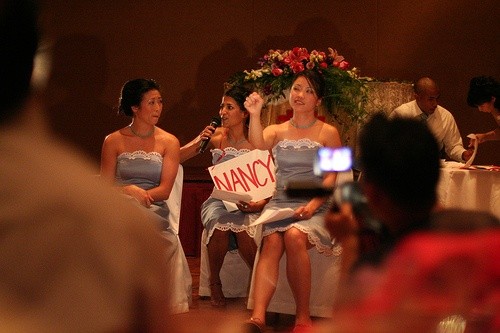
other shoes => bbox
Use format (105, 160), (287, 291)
(243, 318), (263, 333)
(211, 282), (227, 306)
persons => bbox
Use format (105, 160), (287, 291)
(310, 76), (500, 333)
(179, 84), (259, 310)
(0, 0), (192, 333)
(101, 79), (181, 225)
(243, 69), (342, 333)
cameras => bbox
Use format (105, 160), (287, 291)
(318, 147), (369, 215)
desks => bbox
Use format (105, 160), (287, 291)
(435, 161), (500, 222)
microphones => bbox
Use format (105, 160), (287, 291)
(198, 116), (221, 153)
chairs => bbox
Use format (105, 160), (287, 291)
(162, 163), (193, 315)
(247, 167), (362, 319)
(198, 228), (261, 298)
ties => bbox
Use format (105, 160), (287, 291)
(421, 113), (429, 121)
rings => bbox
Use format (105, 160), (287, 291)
(300, 213), (303, 217)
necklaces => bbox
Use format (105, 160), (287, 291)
(225, 128), (250, 147)
(290, 116), (318, 128)
(129, 124), (157, 138)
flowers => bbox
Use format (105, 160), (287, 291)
(227, 46), (373, 126)
